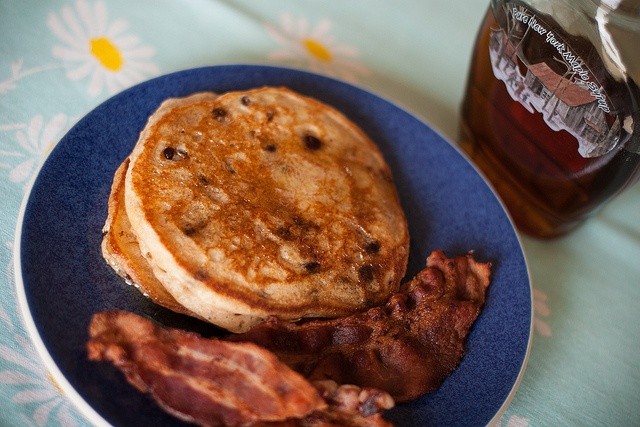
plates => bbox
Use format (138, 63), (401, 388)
(14, 65), (534, 426)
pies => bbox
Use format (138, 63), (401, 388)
(102, 88), (410, 333)
